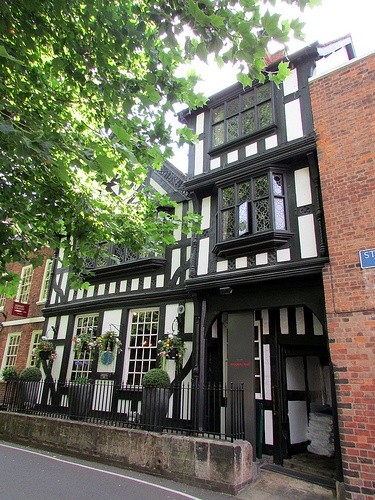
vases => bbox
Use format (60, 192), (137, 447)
(165, 347), (179, 360)
(81, 343), (89, 353)
(39, 351), (51, 360)
(103, 342), (114, 352)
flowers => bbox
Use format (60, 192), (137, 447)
(93, 331), (123, 355)
(30, 335), (57, 362)
(156, 334), (185, 361)
(71, 332), (94, 366)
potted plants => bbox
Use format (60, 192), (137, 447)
(66, 375), (92, 421)
(0, 366), (20, 408)
(139, 368), (172, 433)
(17, 366), (42, 411)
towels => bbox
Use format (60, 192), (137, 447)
(305, 411), (335, 457)
